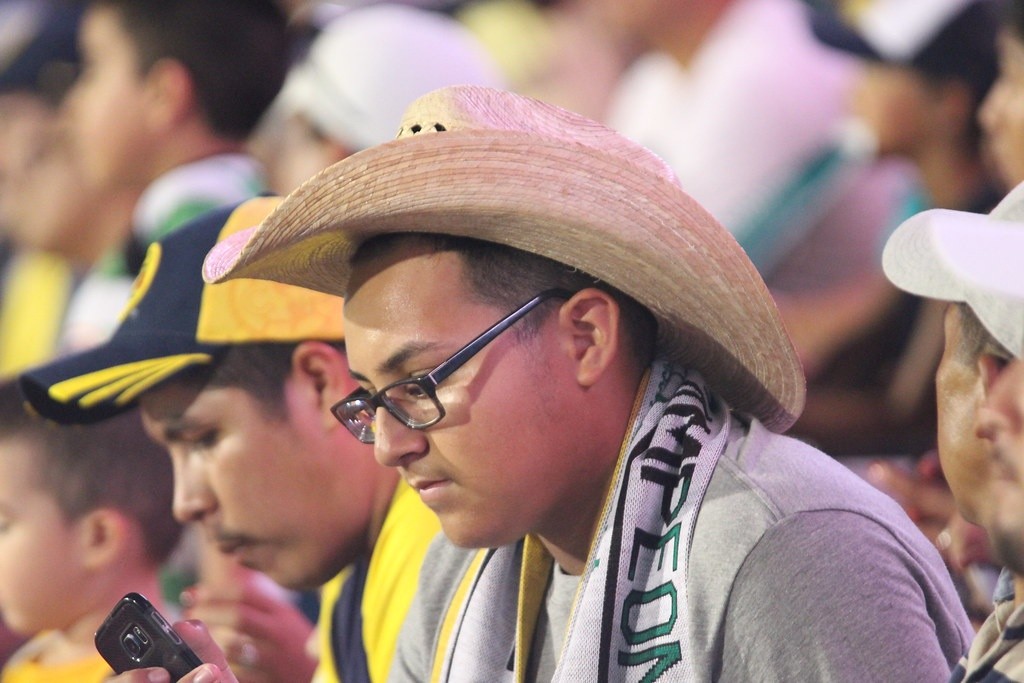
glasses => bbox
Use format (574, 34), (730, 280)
(329, 288), (581, 445)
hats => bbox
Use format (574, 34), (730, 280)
(881, 180), (1023, 359)
(201, 85), (806, 434)
(17, 193), (346, 424)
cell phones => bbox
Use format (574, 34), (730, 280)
(95, 591), (203, 683)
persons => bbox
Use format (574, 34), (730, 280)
(203, 86), (976, 683)
(0, 0), (1024, 683)
